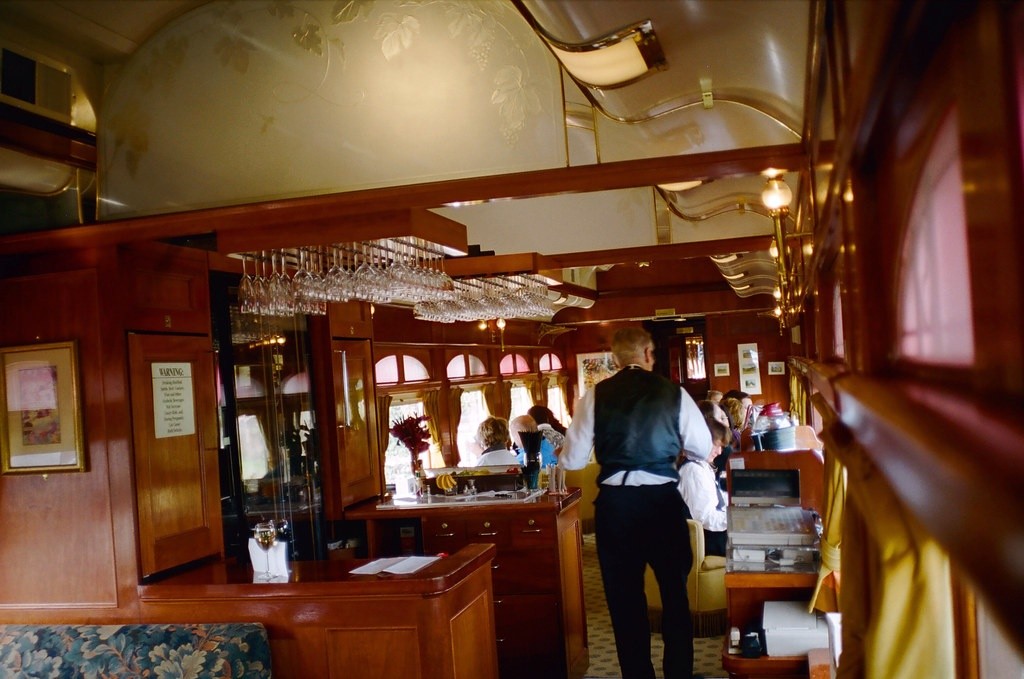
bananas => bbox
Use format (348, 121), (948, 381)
(436, 473), (457, 491)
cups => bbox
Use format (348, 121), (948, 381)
(445, 476), (458, 496)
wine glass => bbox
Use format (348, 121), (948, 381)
(254, 522), (279, 580)
(237, 237), (557, 324)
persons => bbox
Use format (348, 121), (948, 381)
(677, 389), (753, 558)
(553, 328), (713, 679)
(475, 405), (568, 468)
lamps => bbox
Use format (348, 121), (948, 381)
(760, 173), (793, 211)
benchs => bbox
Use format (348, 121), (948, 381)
(0, 619), (274, 679)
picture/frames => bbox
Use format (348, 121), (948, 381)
(0, 337), (87, 476)
(768, 362), (785, 375)
(576, 352), (622, 400)
(737, 342), (763, 395)
(713, 362), (730, 377)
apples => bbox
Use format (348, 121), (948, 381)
(507, 467), (518, 473)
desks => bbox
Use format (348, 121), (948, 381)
(724, 509), (827, 637)
(725, 425), (826, 516)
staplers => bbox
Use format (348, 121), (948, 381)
(728, 625), (742, 656)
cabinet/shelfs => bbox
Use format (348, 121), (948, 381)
(346, 485), (591, 679)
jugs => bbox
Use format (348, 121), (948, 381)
(743, 401), (795, 451)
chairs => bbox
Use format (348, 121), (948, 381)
(642, 518), (730, 637)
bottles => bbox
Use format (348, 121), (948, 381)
(414, 470), (423, 499)
(417, 459), (427, 496)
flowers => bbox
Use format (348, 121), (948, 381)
(389, 411), (434, 476)
